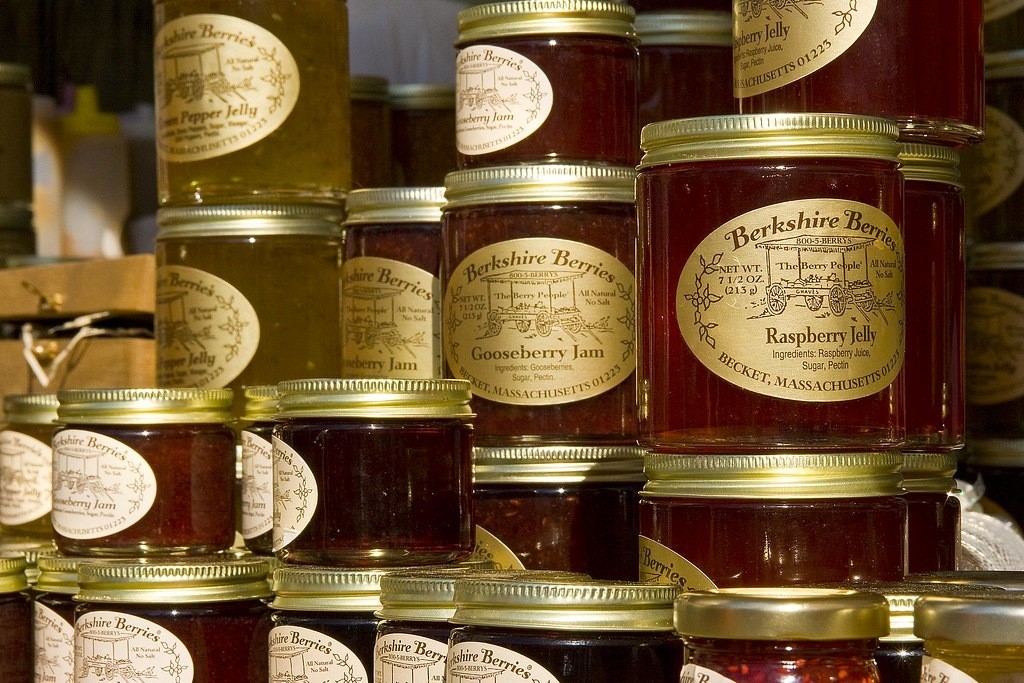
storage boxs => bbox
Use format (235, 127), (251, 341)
(0, 253), (155, 394)
